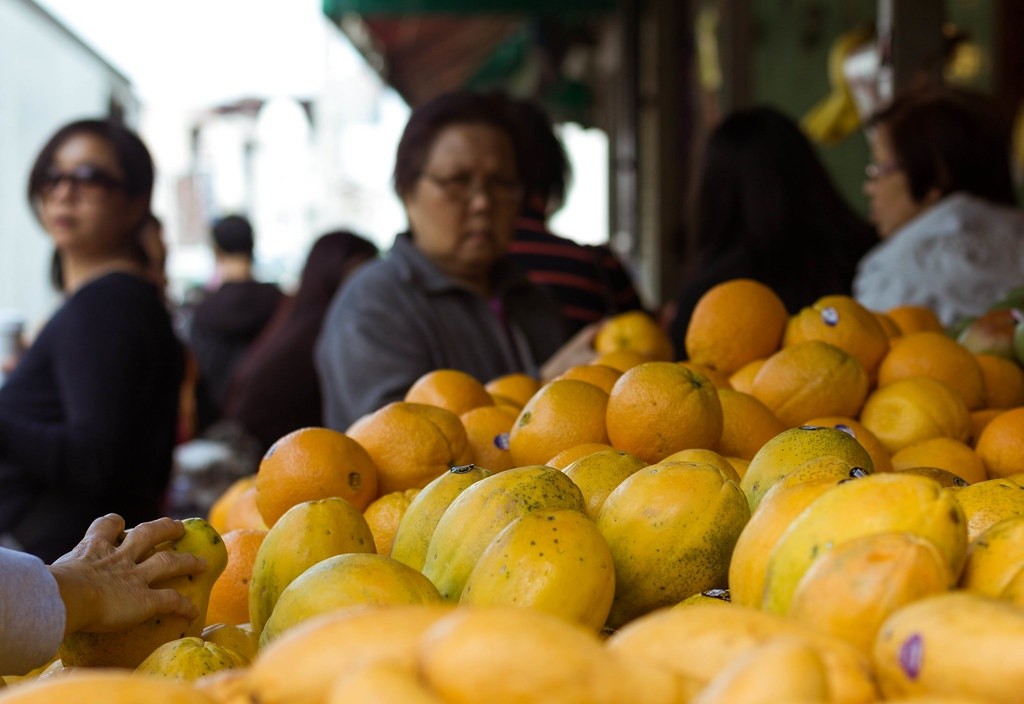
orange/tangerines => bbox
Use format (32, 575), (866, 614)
(205, 279), (1024, 632)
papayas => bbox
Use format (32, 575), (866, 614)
(0, 426), (1024, 704)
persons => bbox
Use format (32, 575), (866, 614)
(312, 91), (645, 432)
(197, 215), (288, 436)
(661, 107), (874, 355)
(0, 121), (197, 566)
(227, 231), (376, 470)
(855, 91), (1024, 323)
(0, 514), (200, 678)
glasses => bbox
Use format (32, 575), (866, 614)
(419, 171), (522, 206)
(41, 164), (125, 192)
